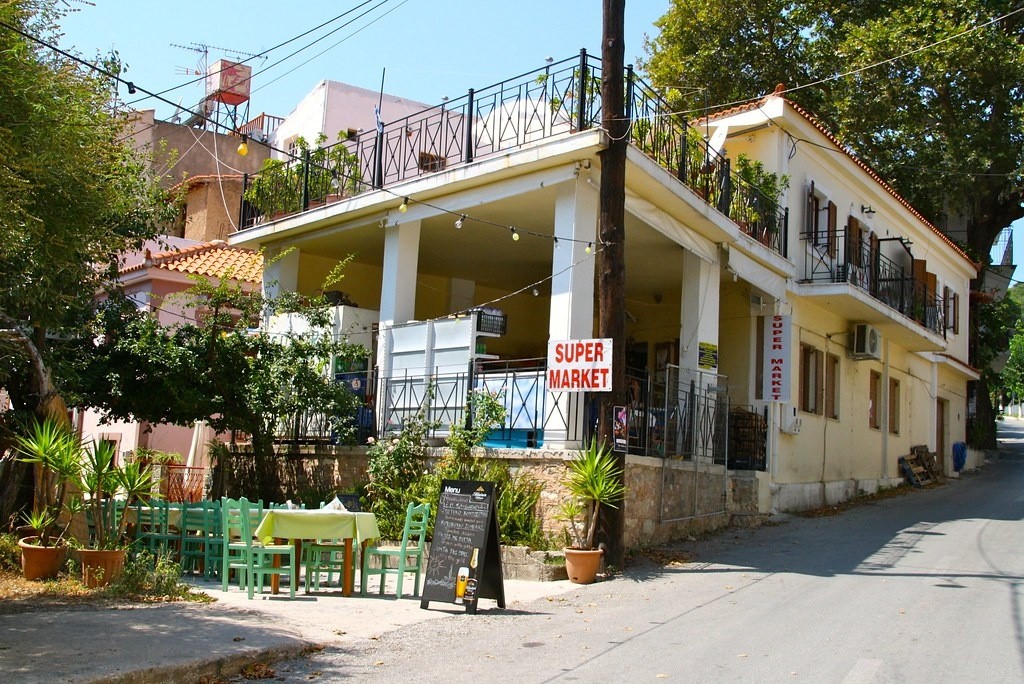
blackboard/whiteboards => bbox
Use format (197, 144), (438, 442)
(420, 479), (505, 616)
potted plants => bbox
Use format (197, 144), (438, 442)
(11, 409), (96, 582)
(551, 435), (631, 583)
(240, 131), (366, 222)
(535, 68), (791, 249)
(69, 432), (167, 588)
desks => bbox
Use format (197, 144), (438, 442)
(253, 507), (380, 596)
(84, 505), (274, 580)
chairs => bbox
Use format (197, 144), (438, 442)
(89, 498), (356, 599)
(361, 502), (431, 598)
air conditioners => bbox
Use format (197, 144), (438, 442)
(854, 324), (882, 360)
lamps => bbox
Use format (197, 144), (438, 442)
(861, 204), (876, 219)
